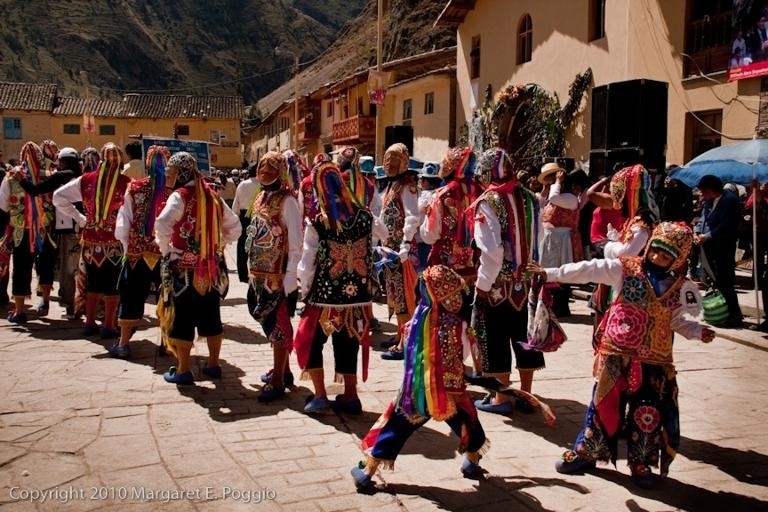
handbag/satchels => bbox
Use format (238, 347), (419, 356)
(704, 291), (729, 324)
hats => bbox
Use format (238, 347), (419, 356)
(419, 161), (443, 180)
(539, 163), (565, 184)
(359, 156), (378, 176)
(60, 147), (78, 158)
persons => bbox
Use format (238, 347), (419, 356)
(0, 140), (768, 494)
(697, 0), (768, 67)
(306, 109), (314, 132)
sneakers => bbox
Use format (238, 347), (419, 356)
(556, 452), (596, 474)
(475, 394), (511, 413)
(165, 366), (193, 383)
(351, 461), (371, 488)
(303, 397), (328, 411)
(83, 323), (131, 359)
(336, 394), (362, 412)
(201, 363), (220, 377)
(382, 337), (403, 359)
(7, 310), (26, 322)
(257, 373), (292, 400)
(461, 457), (477, 474)
